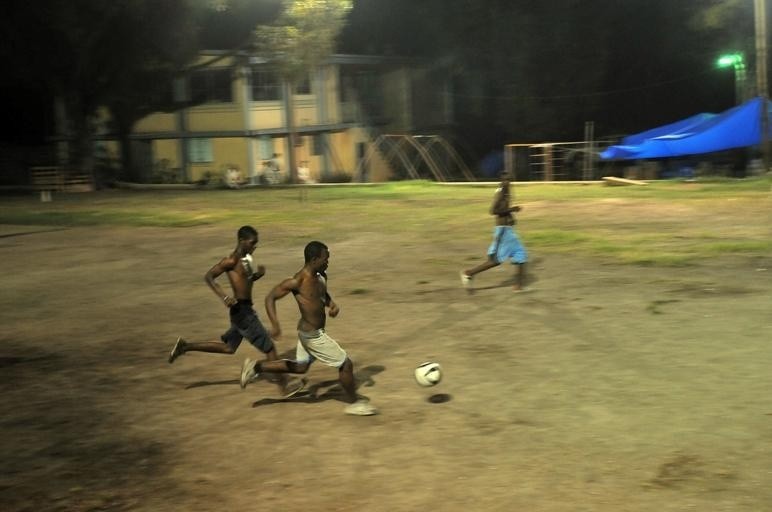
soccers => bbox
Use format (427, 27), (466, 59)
(414, 361), (441, 388)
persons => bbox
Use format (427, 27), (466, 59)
(240, 240), (377, 416)
(459, 170), (528, 295)
(296, 160), (311, 204)
(168, 225), (308, 399)
(268, 153), (282, 184)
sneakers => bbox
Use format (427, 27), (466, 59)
(512, 286), (533, 295)
(241, 358), (260, 389)
(167, 336), (185, 363)
(343, 401), (376, 416)
(461, 271), (472, 289)
(280, 382), (304, 400)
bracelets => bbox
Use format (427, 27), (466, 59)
(223, 295), (231, 304)
